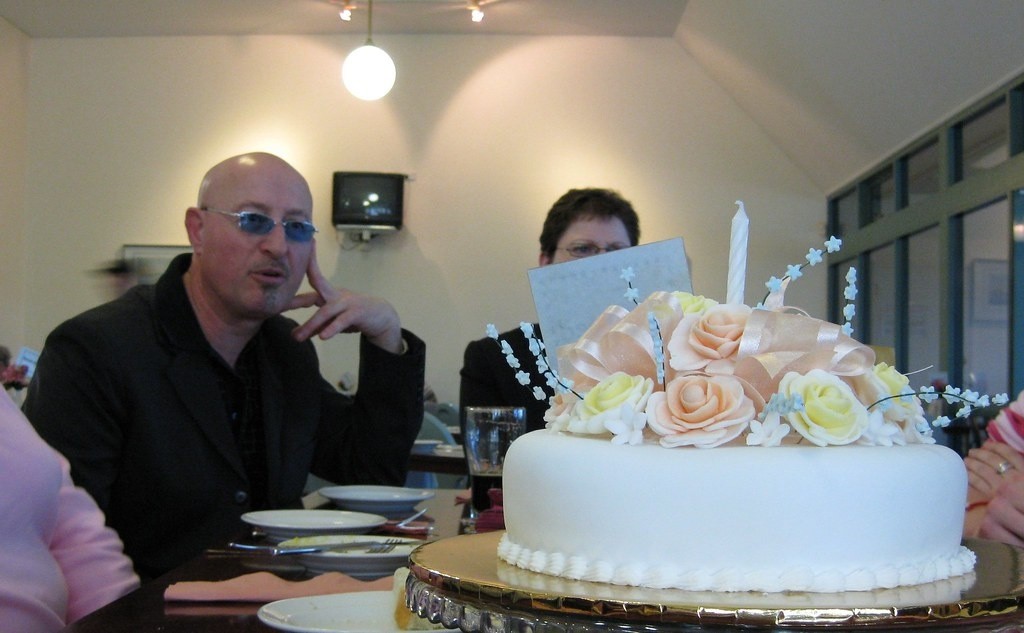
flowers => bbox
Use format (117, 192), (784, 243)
(485, 236), (1010, 446)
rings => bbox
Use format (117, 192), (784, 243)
(997, 461), (1012, 473)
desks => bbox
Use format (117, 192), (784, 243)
(57, 471), (1023, 633)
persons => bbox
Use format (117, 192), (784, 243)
(104, 258), (144, 296)
(16, 147), (428, 583)
(456, 188), (640, 439)
(422, 383), (461, 426)
(963, 387), (1024, 547)
(0, 380), (162, 633)
(0, 346), (30, 408)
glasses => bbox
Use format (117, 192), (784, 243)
(557, 238), (628, 258)
(202, 207), (315, 243)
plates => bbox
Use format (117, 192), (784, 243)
(412, 440), (442, 455)
(318, 485), (435, 517)
(277, 535), (431, 575)
(257, 591), (463, 633)
(241, 510), (387, 540)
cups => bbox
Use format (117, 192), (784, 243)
(465, 407), (526, 517)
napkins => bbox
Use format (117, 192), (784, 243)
(161, 572), (395, 604)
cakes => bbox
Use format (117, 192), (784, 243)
(486, 233), (1009, 593)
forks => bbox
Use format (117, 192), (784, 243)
(229, 539), (402, 554)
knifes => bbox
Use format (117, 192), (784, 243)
(273, 541), (432, 555)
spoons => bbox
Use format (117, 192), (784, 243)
(394, 508), (428, 527)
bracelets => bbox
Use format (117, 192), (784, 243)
(965, 498), (987, 511)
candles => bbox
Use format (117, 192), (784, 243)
(726, 197), (749, 312)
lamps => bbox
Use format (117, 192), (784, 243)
(340, 1), (395, 101)
